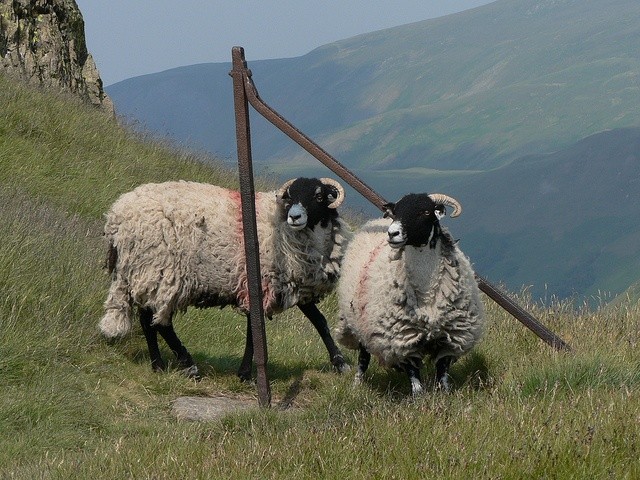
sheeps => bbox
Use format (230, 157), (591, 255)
(99, 179), (351, 380)
(337, 194), (484, 394)
